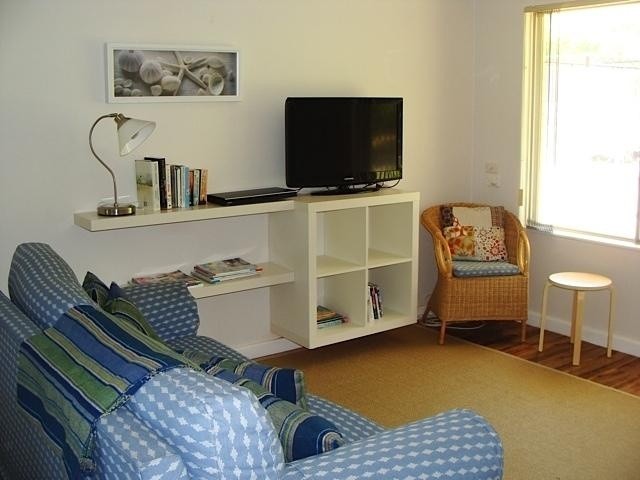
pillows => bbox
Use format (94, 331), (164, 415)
(442, 226), (508, 262)
(176, 349), (310, 412)
(200, 362), (345, 463)
(82, 271), (109, 307)
(104, 281), (165, 346)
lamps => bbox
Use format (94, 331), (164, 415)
(89, 113), (157, 216)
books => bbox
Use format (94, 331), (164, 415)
(135, 157), (209, 210)
(128, 258), (263, 289)
(368, 282), (384, 322)
(317, 306), (348, 328)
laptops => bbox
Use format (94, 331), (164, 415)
(207, 186), (297, 206)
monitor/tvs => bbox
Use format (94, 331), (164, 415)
(285, 97), (403, 196)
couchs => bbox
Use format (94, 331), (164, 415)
(0, 241), (503, 480)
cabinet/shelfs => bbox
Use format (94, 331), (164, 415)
(73, 186), (420, 349)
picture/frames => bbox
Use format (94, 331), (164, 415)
(107, 43), (241, 103)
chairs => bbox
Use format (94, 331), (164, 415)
(419, 202), (530, 345)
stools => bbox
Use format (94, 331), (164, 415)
(538, 272), (616, 366)
